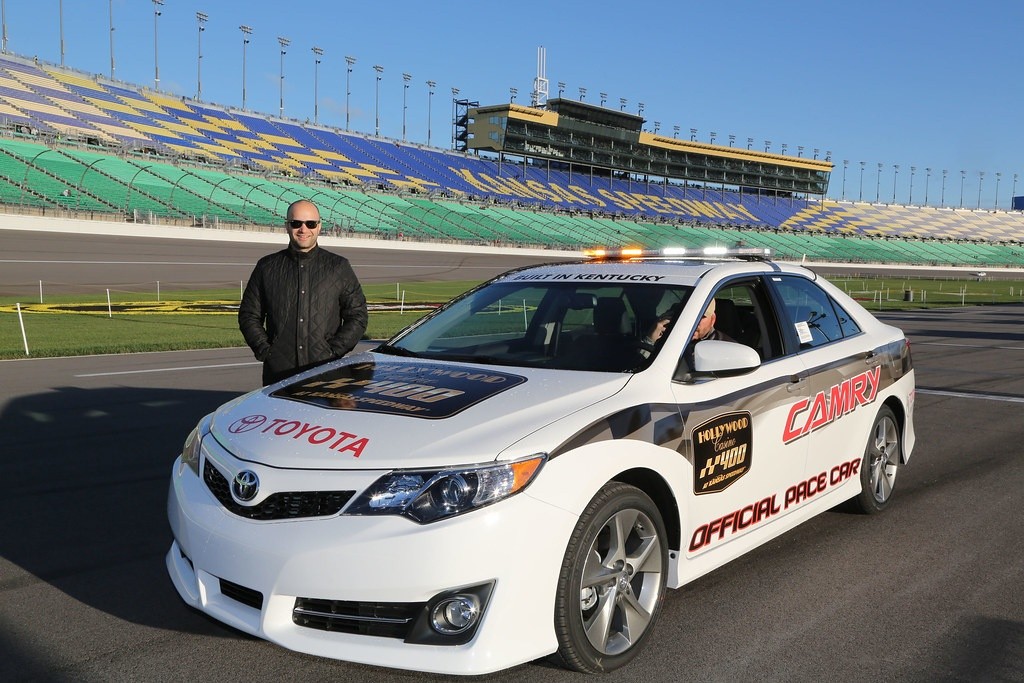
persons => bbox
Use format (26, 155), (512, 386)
(634, 292), (738, 373)
(238, 200), (369, 387)
(273, 361), (375, 408)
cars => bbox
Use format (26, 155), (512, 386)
(167, 247), (917, 675)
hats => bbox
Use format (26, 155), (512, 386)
(704, 298), (715, 317)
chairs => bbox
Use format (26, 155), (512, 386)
(573, 297), (640, 364)
(713, 298), (744, 344)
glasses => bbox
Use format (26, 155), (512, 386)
(287, 219), (320, 229)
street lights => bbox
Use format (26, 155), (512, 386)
(813, 149), (818, 158)
(600, 92), (608, 106)
(638, 102), (644, 116)
(877, 163), (883, 204)
(509, 87), (518, 104)
(557, 81), (566, 98)
(910, 167), (915, 204)
(893, 164), (900, 204)
(729, 135), (735, 146)
(344, 55), (357, 128)
(673, 125), (680, 139)
(995, 173), (1002, 209)
(826, 151), (831, 162)
(655, 121), (660, 134)
(765, 141), (772, 152)
(782, 144), (788, 153)
(860, 161), (865, 201)
(797, 145), (804, 156)
(452, 87), (460, 150)
(841, 159), (849, 199)
(578, 87), (586, 102)
(710, 132), (717, 144)
(278, 35), (290, 119)
(977, 171), (985, 210)
(531, 92), (537, 108)
(374, 65), (383, 136)
(941, 169), (948, 207)
(153, 1), (164, 91)
(239, 24), (253, 111)
(426, 80), (436, 146)
(402, 73), (412, 141)
(620, 98), (628, 111)
(925, 169), (932, 205)
(1011, 173), (1017, 210)
(960, 169), (966, 207)
(312, 46), (322, 124)
(747, 137), (753, 148)
(196, 10), (209, 101)
(690, 128), (697, 141)
